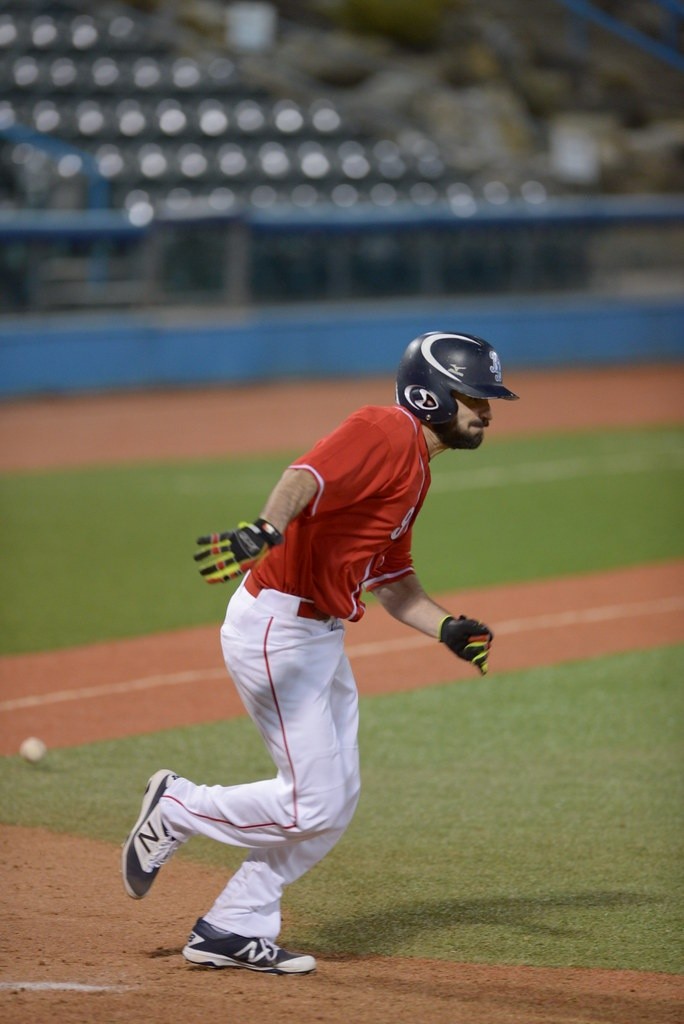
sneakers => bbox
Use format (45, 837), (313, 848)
(121, 769), (183, 900)
(181, 916), (318, 977)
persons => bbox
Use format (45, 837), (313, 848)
(121, 330), (520, 975)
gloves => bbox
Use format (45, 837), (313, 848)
(437, 614), (493, 677)
(193, 518), (285, 586)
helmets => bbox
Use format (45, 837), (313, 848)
(395, 331), (521, 424)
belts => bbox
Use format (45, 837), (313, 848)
(243, 570), (332, 622)
